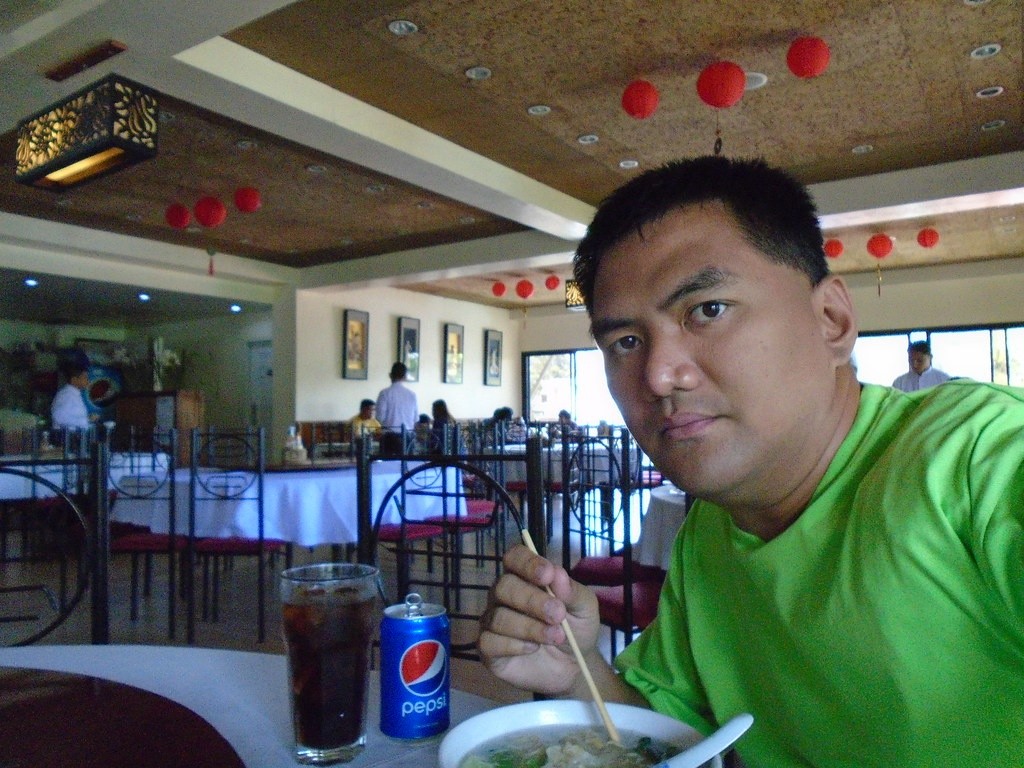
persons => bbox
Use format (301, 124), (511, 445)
(50, 363), (100, 445)
(480, 155), (1024, 768)
(484, 408), (612, 447)
(893, 341), (951, 393)
(350, 362), (460, 456)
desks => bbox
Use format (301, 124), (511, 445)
(113, 455), (462, 628)
(631, 488), (690, 570)
(0, 453), (169, 561)
(481, 437), (638, 535)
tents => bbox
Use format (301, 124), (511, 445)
(868, 232), (892, 298)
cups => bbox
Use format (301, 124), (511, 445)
(278, 562), (385, 768)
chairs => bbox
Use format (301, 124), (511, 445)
(0, 413), (647, 701)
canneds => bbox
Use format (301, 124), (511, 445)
(379, 593), (452, 741)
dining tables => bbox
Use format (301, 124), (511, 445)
(0, 646), (495, 768)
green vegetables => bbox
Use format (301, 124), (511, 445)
(633, 736), (682, 762)
(489, 748), (547, 768)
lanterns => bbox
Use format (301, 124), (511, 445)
(517, 280), (532, 317)
(917, 229), (938, 248)
(193, 197), (228, 277)
(492, 283), (506, 297)
(696, 58), (746, 155)
(622, 80), (657, 121)
(824, 239), (843, 257)
(545, 276), (560, 289)
(166, 203), (190, 231)
(786, 37), (830, 80)
(234, 187), (262, 213)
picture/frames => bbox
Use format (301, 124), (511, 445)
(443, 323), (464, 384)
(398, 316), (421, 382)
(342, 309), (369, 380)
(483, 330), (503, 385)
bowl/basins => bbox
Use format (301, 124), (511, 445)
(438, 699), (724, 768)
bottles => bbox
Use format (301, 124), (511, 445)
(40, 432), (49, 452)
(284, 427), (297, 451)
